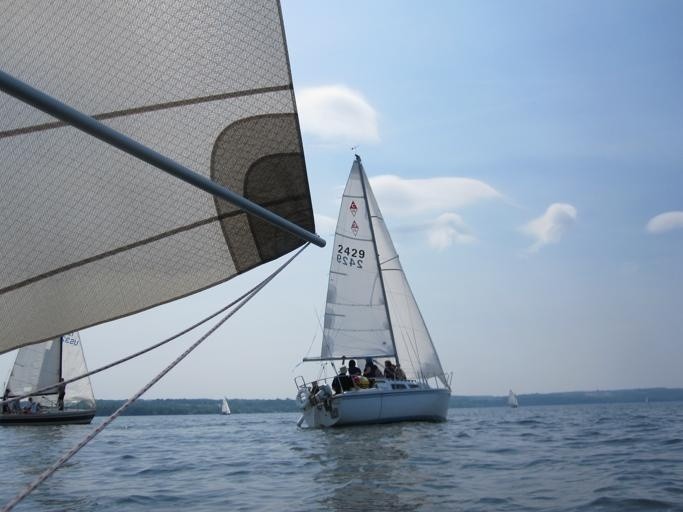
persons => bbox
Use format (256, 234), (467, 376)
(350, 372), (370, 389)
(382, 359), (398, 390)
(11, 398), (22, 413)
(2, 388), (11, 414)
(57, 377), (65, 410)
(348, 359), (362, 376)
(331, 365), (354, 394)
(24, 397), (38, 412)
(362, 357), (383, 387)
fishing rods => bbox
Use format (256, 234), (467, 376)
(314, 305), (346, 393)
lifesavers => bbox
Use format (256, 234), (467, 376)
(296, 388), (311, 408)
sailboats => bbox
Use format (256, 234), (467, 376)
(507, 389), (519, 407)
(1, 1), (323, 509)
(290, 148), (455, 426)
(219, 397), (231, 415)
(2, 325), (102, 429)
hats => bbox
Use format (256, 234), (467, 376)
(337, 366), (349, 374)
